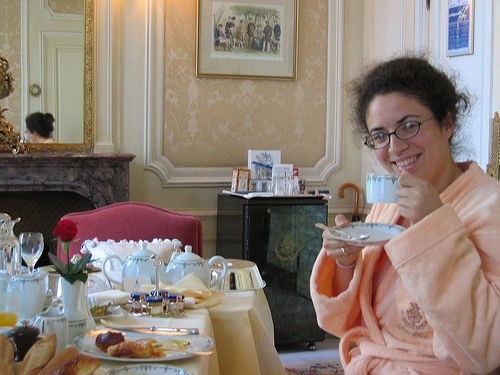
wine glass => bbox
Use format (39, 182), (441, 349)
(19, 232), (44, 276)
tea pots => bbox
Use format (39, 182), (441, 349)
(32, 304), (69, 354)
(160, 244), (227, 289)
(101, 242), (166, 294)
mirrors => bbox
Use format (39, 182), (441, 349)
(0, 0), (95, 153)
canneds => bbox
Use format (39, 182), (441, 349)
(165, 294), (185, 313)
(131, 291), (149, 313)
(150, 289), (168, 310)
(146, 296), (163, 314)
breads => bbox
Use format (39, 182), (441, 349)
(0, 333), (102, 375)
(95, 331), (167, 359)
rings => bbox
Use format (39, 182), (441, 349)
(341, 246), (347, 256)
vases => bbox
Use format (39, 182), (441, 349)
(61, 277), (87, 322)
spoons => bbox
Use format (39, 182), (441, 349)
(316, 223), (371, 241)
(100, 319), (197, 336)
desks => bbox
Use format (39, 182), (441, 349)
(84, 258), (287, 375)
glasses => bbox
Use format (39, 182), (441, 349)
(363, 115), (437, 150)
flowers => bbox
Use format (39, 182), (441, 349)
(48, 220), (93, 283)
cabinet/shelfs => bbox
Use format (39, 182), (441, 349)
(216, 193), (329, 351)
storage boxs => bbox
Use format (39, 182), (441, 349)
(230, 168), (249, 193)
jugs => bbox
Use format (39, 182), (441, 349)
(0, 213), (24, 279)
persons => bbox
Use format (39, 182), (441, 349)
(25, 113), (57, 144)
(310, 53), (500, 375)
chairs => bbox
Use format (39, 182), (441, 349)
(58, 202), (203, 264)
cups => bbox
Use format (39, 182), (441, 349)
(366, 174), (399, 204)
(0, 288), (22, 333)
(266, 176), (301, 196)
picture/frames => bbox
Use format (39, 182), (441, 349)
(194, 0), (301, 81)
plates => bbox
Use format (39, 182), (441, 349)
(104, 365), (192, 375)
(327, 223), (405, 246)
(71, 330), (214, 361)
